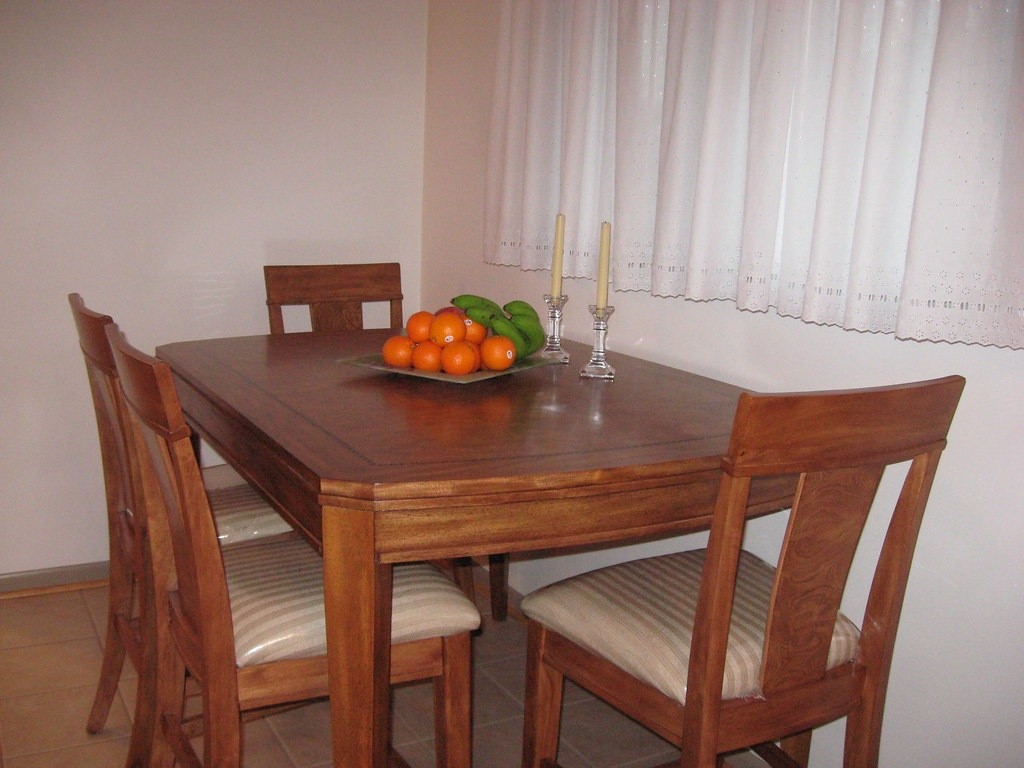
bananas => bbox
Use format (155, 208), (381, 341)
(450, 294), (546, 361)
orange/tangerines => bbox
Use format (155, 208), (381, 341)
(382, 306), (516, 375)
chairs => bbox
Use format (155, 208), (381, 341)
(66, 294), (292, 734)
(262, 263), (402, 331)
(519, 375), (967, 768)
(101, 324), (481, 768)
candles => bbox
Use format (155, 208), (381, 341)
(595, 221), (610, 317)
(552, 214), (563, 305)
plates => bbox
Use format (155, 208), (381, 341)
(336, 353), (560, 384)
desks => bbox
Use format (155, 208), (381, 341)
(156, 330), (802, 768)
